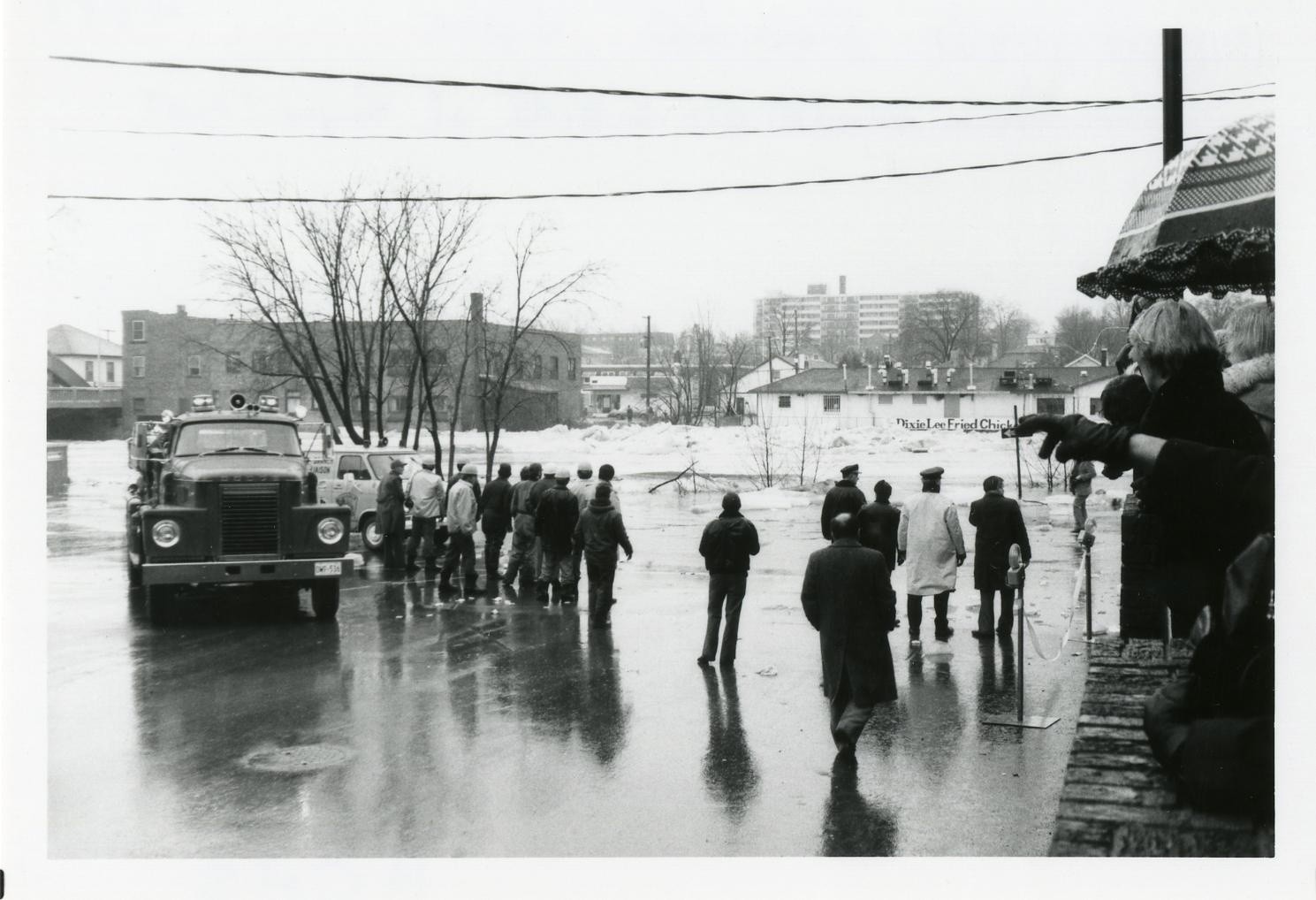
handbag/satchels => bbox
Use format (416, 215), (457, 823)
(405, 497), (413, 508)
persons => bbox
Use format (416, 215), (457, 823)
(857, 480), (901, 629)
(969, 475), (1032, 640)
(697, 491), (760, 667)
(1068, 459), (1096, 534)
(802, 512), (901, 763)
(897, 466), (965, 639)
(377, 458), (632, 629)
(1011, 298), (1275, 811)
(820, 464), (867, 543)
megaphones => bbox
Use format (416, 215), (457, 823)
(230, 394), (246, 410)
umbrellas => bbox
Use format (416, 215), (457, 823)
(1078, 113), (1275, 299)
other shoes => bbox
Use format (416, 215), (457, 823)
(698, 655), (710, 661)
(384, 560), (611, 628)
(997, 627), (1012, 636)
(972, 628), (995, 638)
(909, 626), (921, 634)
(834, 729), (855, 758)
(937, 627), (954, 638)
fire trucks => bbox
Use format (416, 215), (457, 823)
(124, 394), (354, 620)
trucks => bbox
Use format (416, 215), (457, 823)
(310, 444), (450, 552)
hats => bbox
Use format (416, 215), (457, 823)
(161, 410), (174, 419)
(554, 467), (571, 478)
(544, 464), (557, 474)
(920, 466), (944, 481)
(423, 456), (436, 465)
(983, 475), (1004, 491)
(841, 464), (862, 474)
(459, 464), (477, 475)
(578, 462), (593, 471)
(456, 457), (471, 466)
(390, 459), (408, 468)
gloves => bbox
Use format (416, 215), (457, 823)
(1013, 409), (1131, 472)
